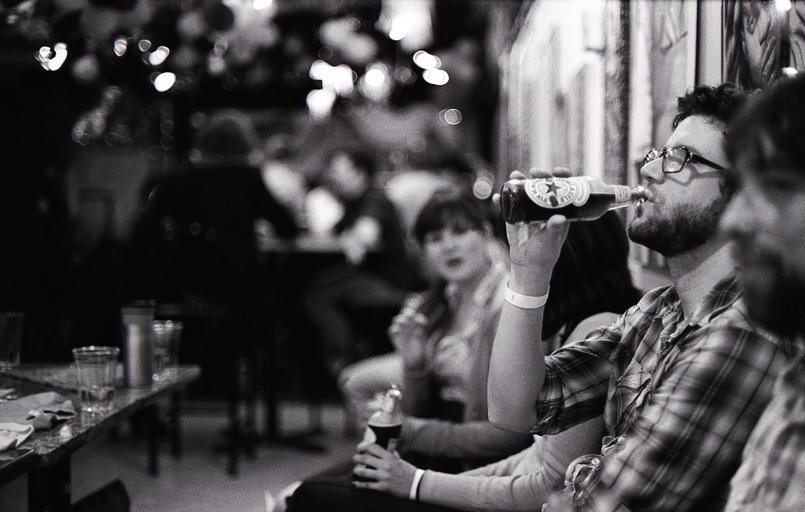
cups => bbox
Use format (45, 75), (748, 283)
(151, 321), (182, 382)
(72, 346), (119, 412)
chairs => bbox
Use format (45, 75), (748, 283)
(320, 301), (405, 449)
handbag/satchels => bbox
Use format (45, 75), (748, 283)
(285, 448), (465, 511)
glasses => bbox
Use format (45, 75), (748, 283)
(641, 146), (728, 174)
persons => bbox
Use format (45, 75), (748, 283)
(274, 73), (804, 512)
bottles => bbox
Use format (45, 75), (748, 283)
(498, 178), (647, 223)
(353, 389), (404, 485)
(120, 301), (155, 388)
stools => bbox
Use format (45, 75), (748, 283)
(155, 298), (258, 477)
